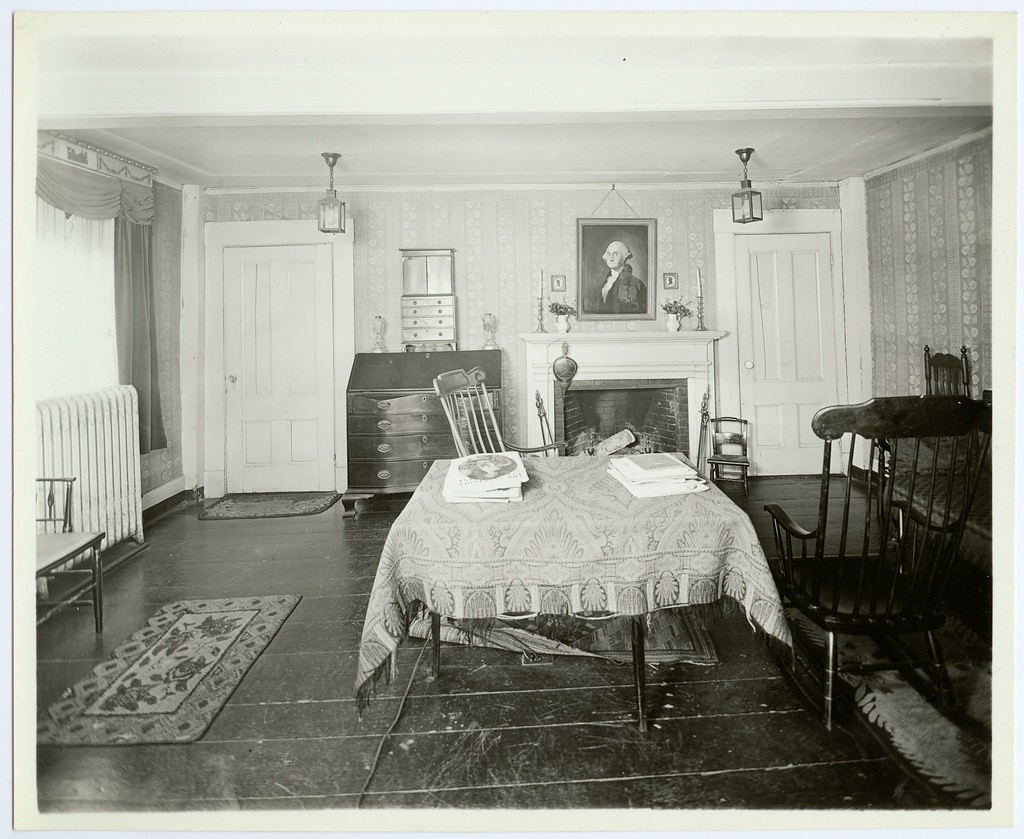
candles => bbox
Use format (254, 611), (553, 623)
(539, 268), (543, 298)
(697, 268), (702, 296)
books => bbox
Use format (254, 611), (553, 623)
(441, 451), (529, 504)
(607, 451), (710, 499)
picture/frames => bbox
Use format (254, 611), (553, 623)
(576, 218), (657, 321)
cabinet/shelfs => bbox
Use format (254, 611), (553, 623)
(399, 248), (459, 351)
(340, 348), (502, 518)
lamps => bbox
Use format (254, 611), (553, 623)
(316, 151), (346, 234)
(731, 148), (763, 224)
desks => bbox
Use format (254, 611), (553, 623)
(352, 451), (795, 719)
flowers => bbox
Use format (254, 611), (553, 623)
(657, 296), (692, 320)
(546, 297), (578, 319)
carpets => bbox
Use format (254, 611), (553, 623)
(199, 491), (343, 519)
(36, 593), (302, 745)
(407, 607), (720, 669)
(759, 554), (992, 809)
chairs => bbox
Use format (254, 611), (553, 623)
(36, 478), (106, 639)
(759, 345), (993, 739)
(432, 367), (569, 457)
(707, 417), (748, 496)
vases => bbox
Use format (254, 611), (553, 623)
(554, 314), (571, 333)
(664, 314), (681, 332)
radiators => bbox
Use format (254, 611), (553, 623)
(36, 385), (145, 597)
